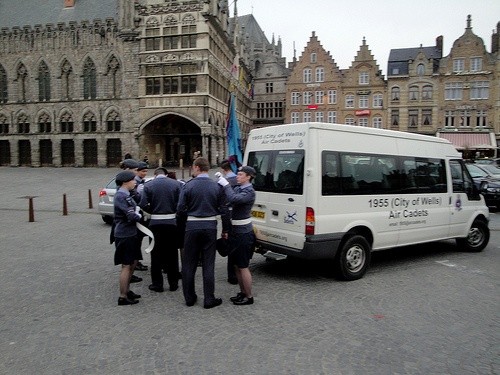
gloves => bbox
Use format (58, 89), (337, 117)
(215, 172), (229, 186)
(137, 184), (144, 193)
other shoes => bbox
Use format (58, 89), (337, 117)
(228, 278), (239, 285)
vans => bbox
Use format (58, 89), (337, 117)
(242, 122), (491, 281)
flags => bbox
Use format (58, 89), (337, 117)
(225, 76), (245, 173)
(233, 65), (256, 99)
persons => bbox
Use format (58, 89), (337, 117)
(143, 167), (184, 291)
(177, 157), (225, 309)
(214, 165), (256, 305)
(220, 154), (242, 239)
(110, 159), (185, 306)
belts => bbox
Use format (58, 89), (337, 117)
(151, 214), (175, 220)
(230, 217), (252, 225)
(136, 222), (154, 254)
(187, 216), (217, 221)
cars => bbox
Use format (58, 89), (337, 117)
(450, 158), (500, 208)
(95, 176), (118, 224)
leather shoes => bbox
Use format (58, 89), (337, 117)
(132, 275), (142, 281)
(231, 293), (245, 301)
(118, 297), (139, 305)
(137, 264), (147, 271)
(187, 294), (198, 306)
(204, 299), (222, 308)
(149, 284), (164, 293)
(235, 297), (254, 305)
(128, 290), (141, 298)
(171, 284), (179, 291)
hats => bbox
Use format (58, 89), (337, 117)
(138, 161), (149, 171)
(116, 171), (136, 186)
(120, 159), (138, 169)
(216, 238), (231, 257)
(237, 166), (256, 178)
(155, 167), (167, 175)
